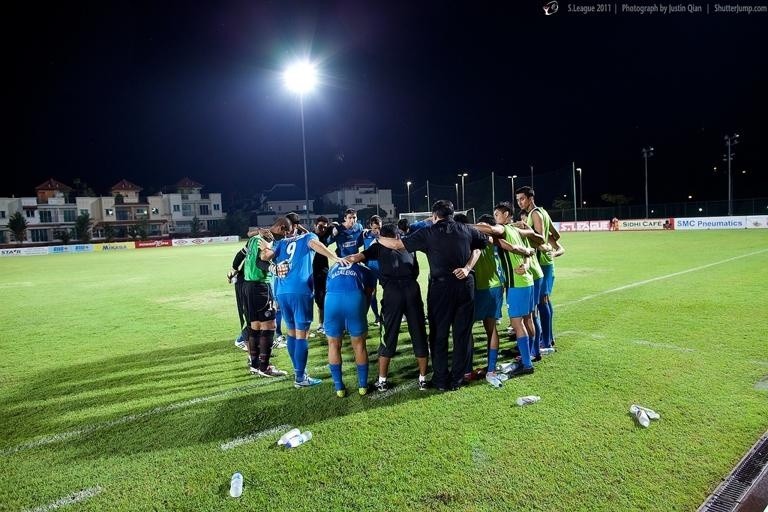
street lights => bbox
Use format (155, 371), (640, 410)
(507, 175), (517, 213)
(455, 183), (459, 210)
(282, 58), (317, 230)
(642, 147), (655, 219)
(575, 167), (583, 209)
(725, 133), (740, 216)
(458, 172), (469, 211)
(406, 181), (412, 212)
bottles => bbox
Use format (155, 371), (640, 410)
(629, 404), (661, 428)
(230, 472), (244, 498)
(276, 427), (313, 449)
(540, 347), (557, 354)
(516, 395), (542, 406)
(486, 362), (519, 388)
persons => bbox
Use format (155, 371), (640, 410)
(227, 185), (565, 394)
(608, 216), (620, 231)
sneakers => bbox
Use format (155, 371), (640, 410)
(294, 372), (323, 389)
(336, 389), (346, 398)
(276, 326), (327, 342)
(272, 340), (286, 349)
(373, 380), (388, 392)
(234, 337), (248, 351)
(358, 383), (370, 396)
(431, 369), (495, 391)
(249, 365), (288, 377)
(496, 315), (555, 375)
(417, 379), (428, 390)
